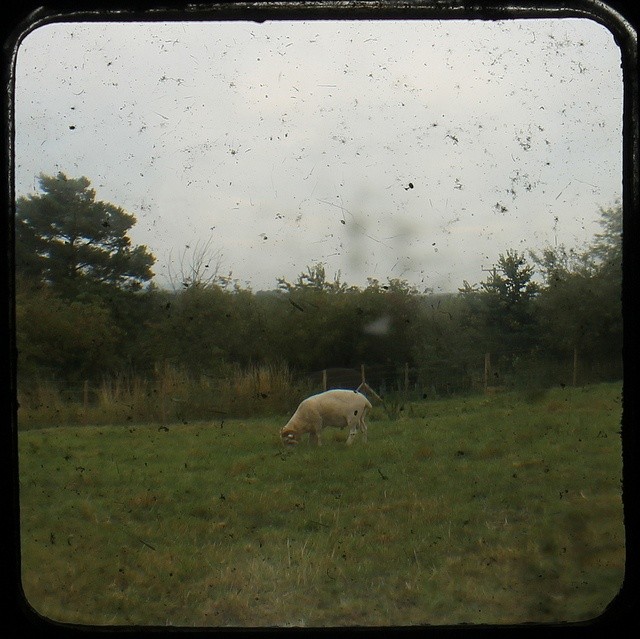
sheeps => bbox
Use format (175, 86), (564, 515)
(281, 389), (371, 448)
